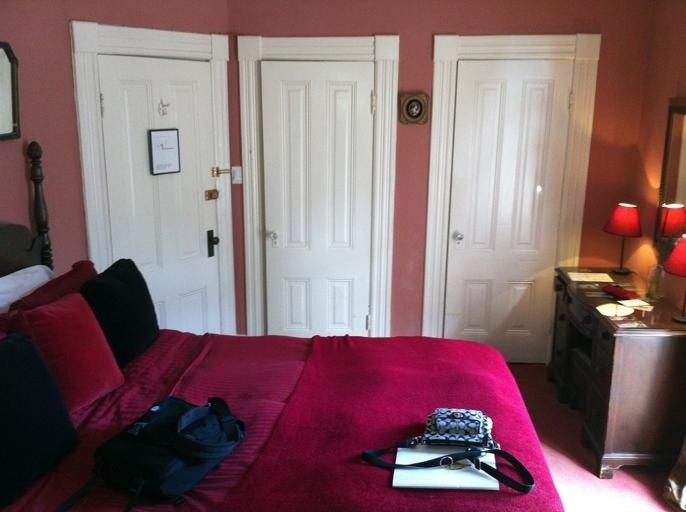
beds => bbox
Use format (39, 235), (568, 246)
(0, 140), (566, 512)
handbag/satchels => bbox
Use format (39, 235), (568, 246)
(91, 395), (246, 506)
(421, 408), (493, 448)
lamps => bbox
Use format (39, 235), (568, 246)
(602, 201), (685, 325)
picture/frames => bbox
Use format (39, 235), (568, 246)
(148, 128), (180, 176)
(0, 40), (21, 139)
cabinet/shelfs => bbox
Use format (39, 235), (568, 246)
(547, 272), (685, 479)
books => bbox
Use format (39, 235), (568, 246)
(391, 446), (500, 490)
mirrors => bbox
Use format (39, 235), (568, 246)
(654, 95), (686, 250)
